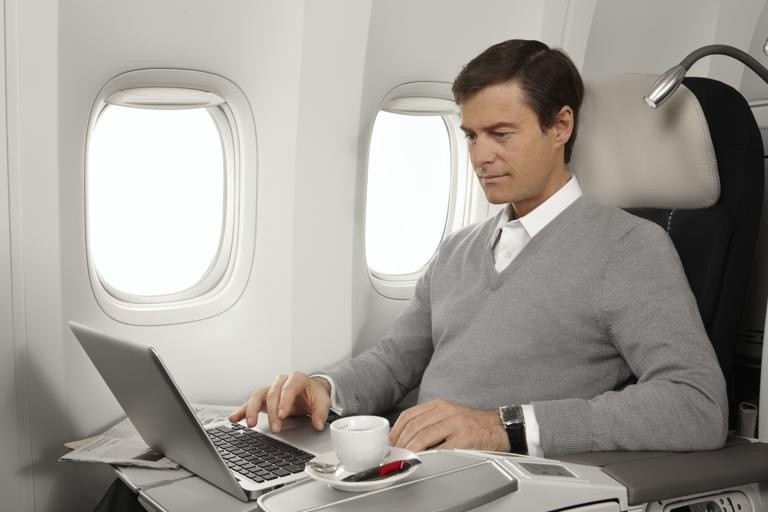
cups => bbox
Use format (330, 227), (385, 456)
(330, 414), (392, 474)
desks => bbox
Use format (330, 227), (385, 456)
(113, 418), (627, 511)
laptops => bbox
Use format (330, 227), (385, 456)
(67, 320), (333, 503)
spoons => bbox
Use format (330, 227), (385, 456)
(312, 450), (391, 475)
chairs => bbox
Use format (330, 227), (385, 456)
(551, 69), (768, 510)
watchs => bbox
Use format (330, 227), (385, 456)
(497, 403), (524, 455)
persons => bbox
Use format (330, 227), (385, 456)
(228, 40), (730, 456)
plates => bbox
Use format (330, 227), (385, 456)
(304, 446), (419, 492)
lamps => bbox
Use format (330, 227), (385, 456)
(644, 43), (767, 109)
